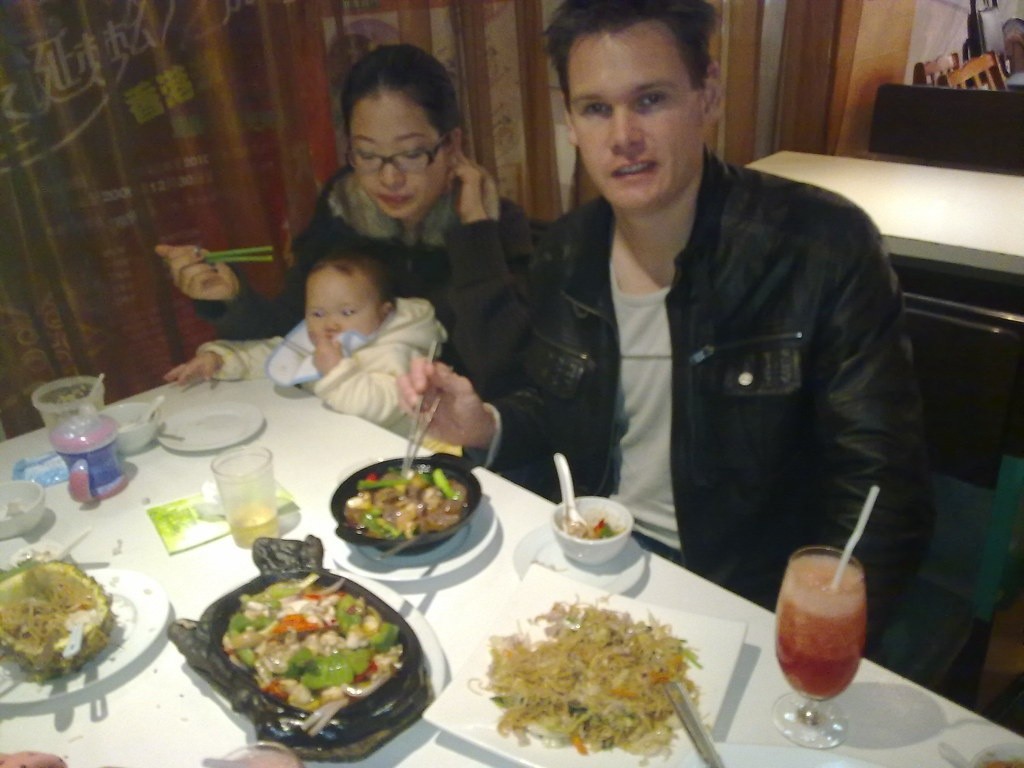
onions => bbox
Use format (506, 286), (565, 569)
(245, 572), (392, 737)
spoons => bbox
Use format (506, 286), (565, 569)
(553, 453), (589, 532)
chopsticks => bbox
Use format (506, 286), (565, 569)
(398, 338), (455, 480)
(199, 245), (277, 269)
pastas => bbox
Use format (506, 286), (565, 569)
(487, 606), (696, 754)
(1, 570), (96, 648)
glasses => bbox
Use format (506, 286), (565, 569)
(345, 132), (450, 175)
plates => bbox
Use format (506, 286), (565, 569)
(421, 563), (747, 768)
(165, 536), (433, 761)
(330, 496), (498, 581)
(155, 398), (265, 452)
(0, 568), (170, 706)
(327, 569), (450, 700)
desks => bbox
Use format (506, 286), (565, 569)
(744, 150), (1024, 295)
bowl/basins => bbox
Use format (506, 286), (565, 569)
(100, 400), (156, 455)
(551, 497), (635, 565)
(328, 450), (482, 557)
(0, 478), (47, 540)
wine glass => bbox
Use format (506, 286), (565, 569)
(770, 546), (867, 751)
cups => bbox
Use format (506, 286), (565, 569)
(32, 375), (127, 504)
(210, 445), (281, 550)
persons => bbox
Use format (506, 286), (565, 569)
(161, 240), (463, 456)
(155, 43), (546, 491)
(396, 0), (940, 655)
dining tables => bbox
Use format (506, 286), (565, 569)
(1, 373), (1024, 768)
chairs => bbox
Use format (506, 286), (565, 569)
(911, 53), (960, 86)
(862, 452), (1024, 697)
(938, 49), (1008, 90)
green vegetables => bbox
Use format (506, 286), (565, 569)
(355, 466), (461, 541)
(226, 587), (400, 691)
